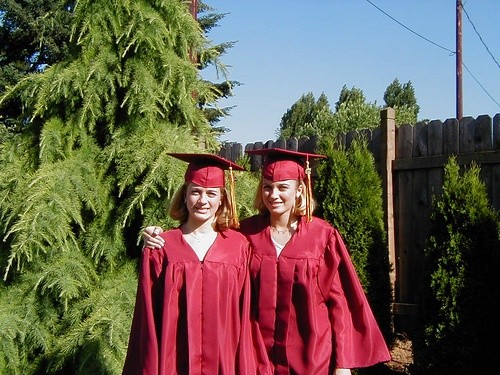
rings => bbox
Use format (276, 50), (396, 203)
(145, 239), (149, 243)
(148, 235), (151, 240)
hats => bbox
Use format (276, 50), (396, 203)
(165, 152), (245, 229)
(244, 147), (329, 223)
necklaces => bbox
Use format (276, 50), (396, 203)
(190, 233), (208, 243)
(271, 226), (296, 234)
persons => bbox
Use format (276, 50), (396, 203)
(121, 152), (273, 375)
(141, 147), (391, 375)
